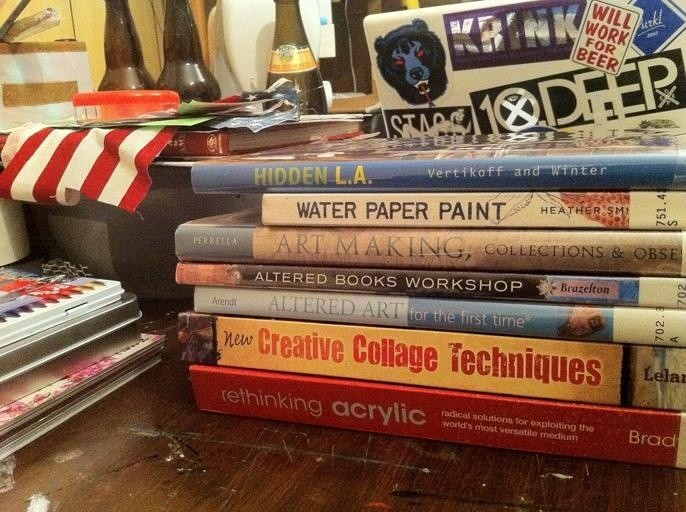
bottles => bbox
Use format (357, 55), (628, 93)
(265, 0), (329, 115)
(156, 0), (222, 103)
(97, 0), (157, 89)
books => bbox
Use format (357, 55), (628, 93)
(0, 0), (686, 472)
